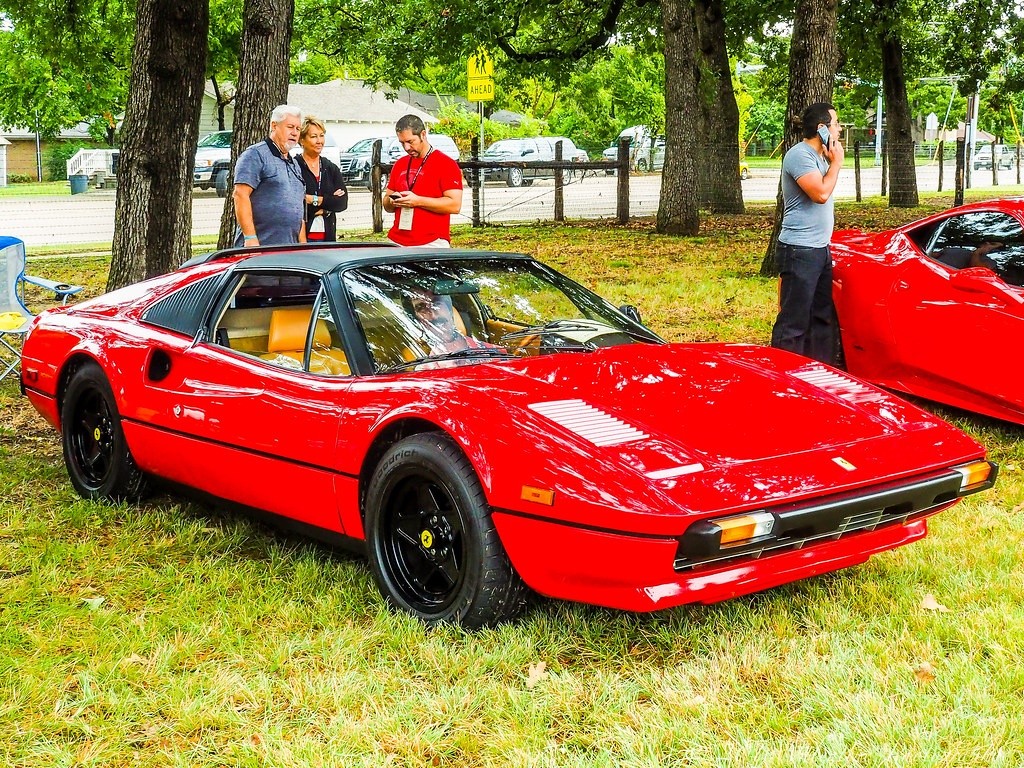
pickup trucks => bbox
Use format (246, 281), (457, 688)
(974, 145), (1014, 170)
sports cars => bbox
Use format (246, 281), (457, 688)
(20, 243), (999, 636)
(777, 197), (1024, 422)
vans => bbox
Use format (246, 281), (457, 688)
(341, 135), (460, 192)
(602, 125), (665, 171)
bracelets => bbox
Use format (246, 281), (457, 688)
(243, 235), (258, 239)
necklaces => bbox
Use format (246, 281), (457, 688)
(302, 154), (320, 177)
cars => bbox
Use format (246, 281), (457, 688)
(462, 138), (574, 187)
(192, 130), (233, 196)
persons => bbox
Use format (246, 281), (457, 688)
(232, 105), (307, 247)
(384, 114), (463, 248)
(294, 114), (348, 242)
(771, 103), (844, 368)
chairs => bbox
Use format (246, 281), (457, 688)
(260, 307), (350, 378)
(0, 235), (84, 381)
(402, 306), (467, 374)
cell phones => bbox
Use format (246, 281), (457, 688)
(388, 195), (401, 200)
(817, 125), (830, 148)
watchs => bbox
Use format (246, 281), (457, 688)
(313, 195), (319, 206)
